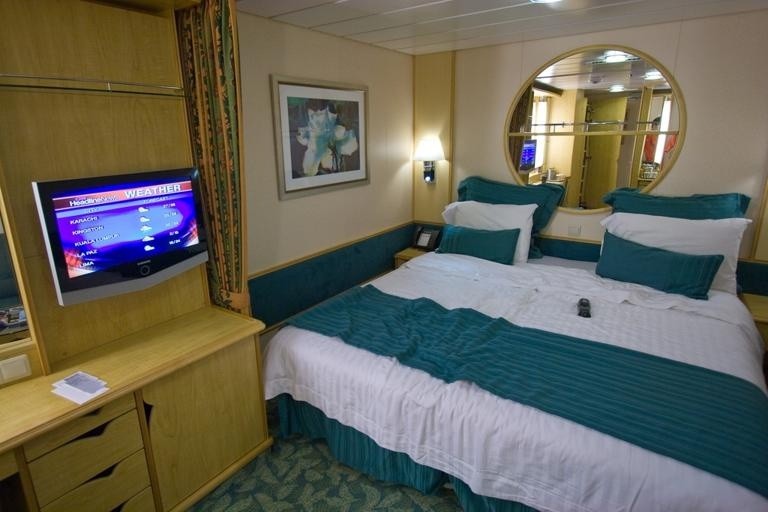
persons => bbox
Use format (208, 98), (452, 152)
(645, 117), (677, 161)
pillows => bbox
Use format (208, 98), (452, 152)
(595, 188), (755, 301)
(435, 176), (566, 265)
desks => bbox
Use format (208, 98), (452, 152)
(1, 302), (277, 512)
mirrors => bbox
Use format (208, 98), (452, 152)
(503, 41), (688, 215)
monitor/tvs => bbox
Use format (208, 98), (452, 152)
(31, 166), (209, 306)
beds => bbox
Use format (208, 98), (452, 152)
(268, 235), (766, 512)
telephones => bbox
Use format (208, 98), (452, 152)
(412, 225), (440, 251)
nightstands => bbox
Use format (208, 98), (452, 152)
(391, 245), (430, 266)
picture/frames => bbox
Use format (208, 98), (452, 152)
(269, 72), (370, 202)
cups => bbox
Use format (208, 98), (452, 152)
(556, 175), (561, 181)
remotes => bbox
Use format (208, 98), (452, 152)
(578, 299), (591, 317)
